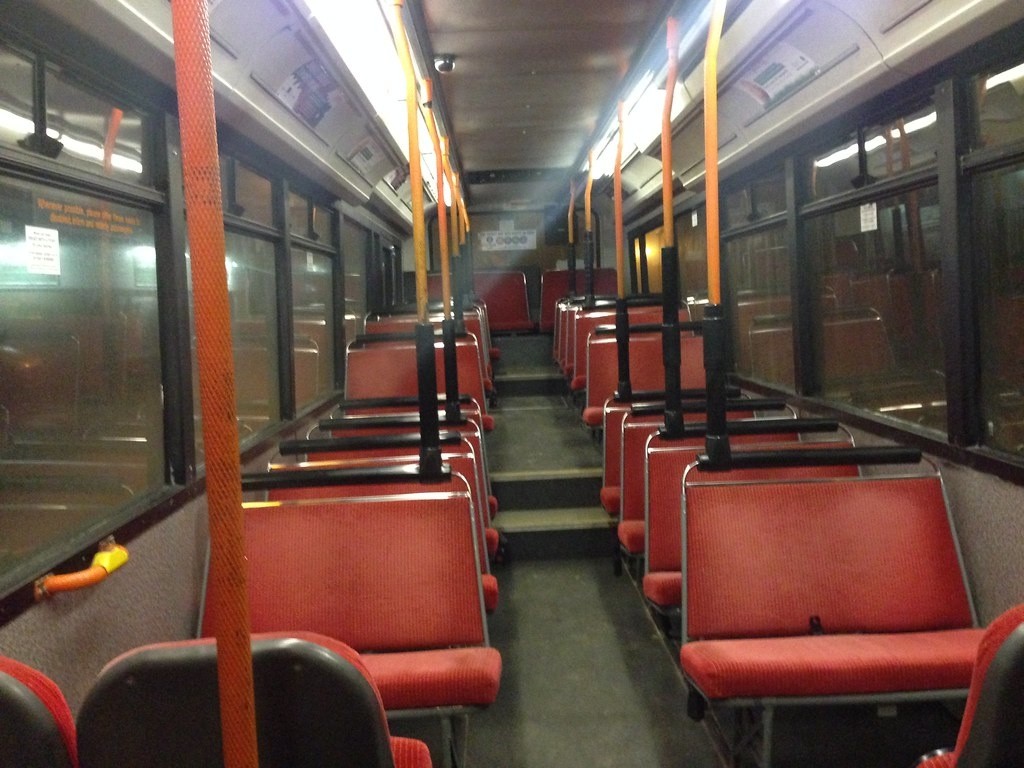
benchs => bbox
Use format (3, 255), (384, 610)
(0, 267), (1024, 768)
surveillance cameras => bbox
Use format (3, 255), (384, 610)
(434, 57), (455, 73)
